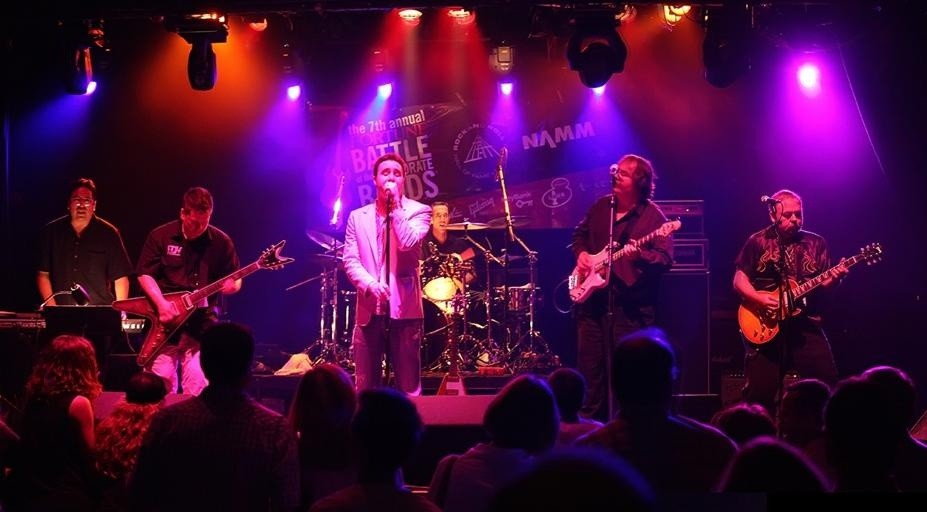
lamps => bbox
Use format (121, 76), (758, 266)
(696, 0), (760, 93)
(562, 1), (628, 91)
(478, 2), (549, 82)
(177, 10), (230, 91)
(51, 18), (109, 94)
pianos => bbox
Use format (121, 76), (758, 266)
(1, 310), (145, 335)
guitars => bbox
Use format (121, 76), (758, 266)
(738, 244), (882, 344)
(568, 219), (682, 303)
(436, 302), (467, 397)
(113, 239), (295, 367)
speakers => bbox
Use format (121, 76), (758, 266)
(249, 373), (303, 415)
(93, 390), (195, 421)
(402, 395), (495, 486)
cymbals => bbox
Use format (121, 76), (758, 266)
(439, 219), (490, 230)
(310, 254), (343, 264)
(491, 215), (532, 228)
(306, 230), (344, 254)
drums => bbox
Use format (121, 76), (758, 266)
(496, 286), (543, 316)
(421, 295), (451, 368)
(419, 254), (462, 302)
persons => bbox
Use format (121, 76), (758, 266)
(419, 200), (478, 273)
(135, 184), (244, 346)
(341, 153), (438, 401)
(569, 156), (675, 418)
(734, 186), (843, 406)
(25, 177), (134, 332)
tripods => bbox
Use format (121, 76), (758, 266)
(286, 225), (357, 372)
(420, 165), (562, 375)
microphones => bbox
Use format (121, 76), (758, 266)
(762, 196), (782, 205)
(494, 147), (507, 182)
(610, 163), (619, 185)
